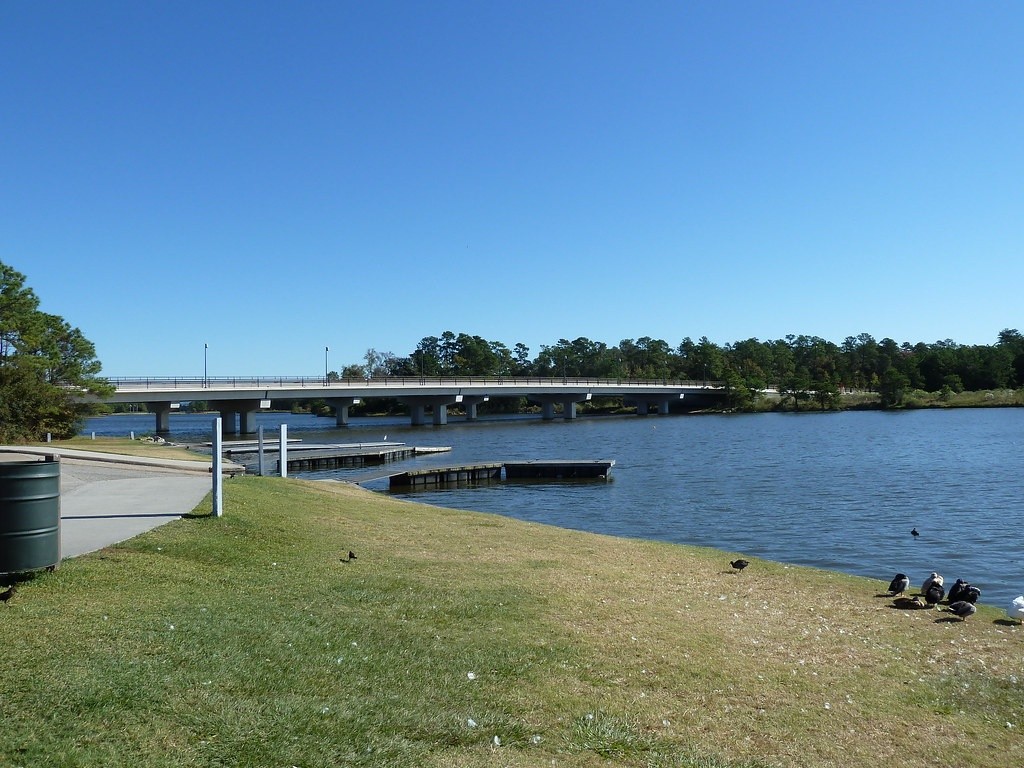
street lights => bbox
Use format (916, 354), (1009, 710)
(702, 363), (708, 386)
(563, 354), (568, 382)
(617, 358), (622, 383)
(324, 347), (329, 385)
(663, 360), (669, 384)
(420, 349), (426, 383)
(204, 343), (209, 387)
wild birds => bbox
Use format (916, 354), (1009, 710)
(348, 551), (357, 561)
(728, 559), (749, 574)
(911, 527), (919, 536)
(887, 572), (981, 622)
(1007, 596), (1024, 625)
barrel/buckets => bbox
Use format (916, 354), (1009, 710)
(0, 461), (59, 575)
